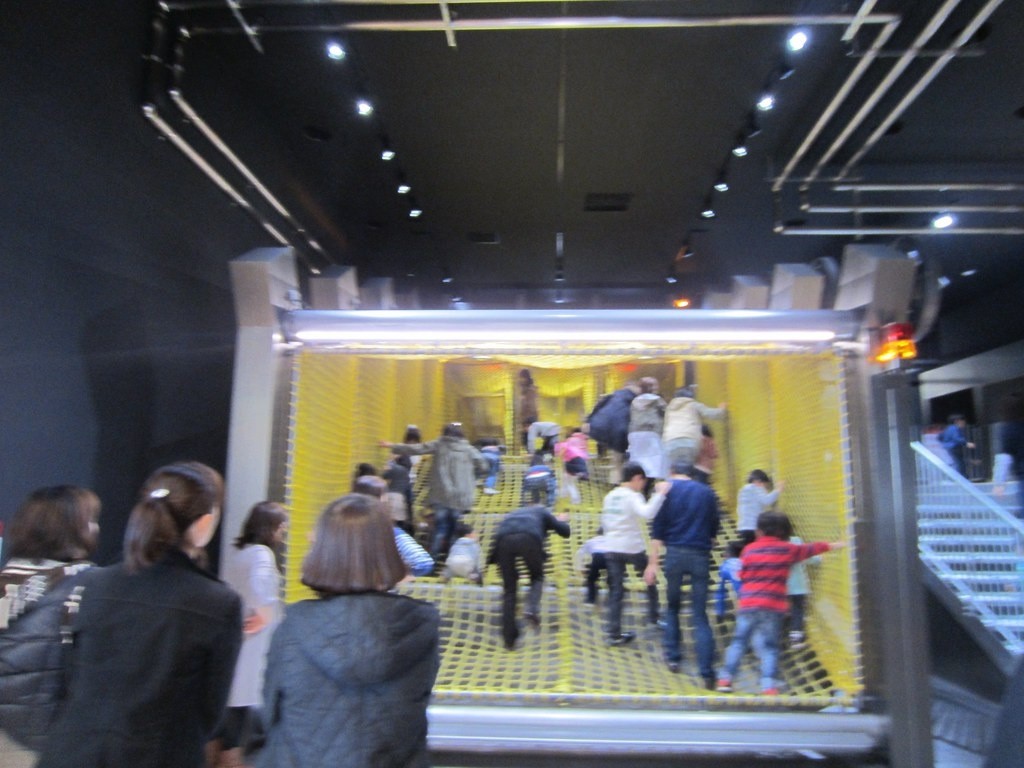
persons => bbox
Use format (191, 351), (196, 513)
(734, 468), (784, 542)
(715, 512), (845, 695)
(922, 414), (977, 481)
(778, 515), (824, 651)
(253, 496), (444, 768)
(202, 500), (288, 768)
(352, 366), (590, 652)
(0, 458), (245, 768)
(713, 540), (743, 624)
(577, 375), (726, 693)
(0, 484), (103, 768)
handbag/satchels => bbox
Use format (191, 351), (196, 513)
(0, 565), (94, 753)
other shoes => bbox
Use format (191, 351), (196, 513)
(607, 632), (636, 645)
(504, 628), (518, 650)
(703, 670), (716, 691)
(762, 686), (778, 695)
(583, 602), (596, 607)
(522, 611), (540, 625)
(642, 617), (667, 629)
(665, 656), (677, 672)
(718, 678), (733, 692)
(789, 630), (804, 649)
(483, 487), (500, 494)
